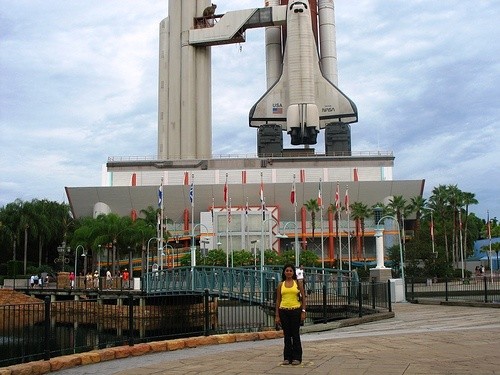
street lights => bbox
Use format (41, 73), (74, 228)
(373, 215), (409, 302)
(282, 221), (299, 269)
(216, 231), (233, 268)
(146, 236), (163, 293)
(260, 217), (283, 303)
(74, 244), (86, 289)
(160, 244), (175, 282)
(191, 223), (212, 292)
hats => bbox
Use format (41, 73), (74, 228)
(94, 270), (98, 274)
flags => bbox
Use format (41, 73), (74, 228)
(157, 172), (350, 232)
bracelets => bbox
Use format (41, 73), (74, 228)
(301, 309), (306, 312)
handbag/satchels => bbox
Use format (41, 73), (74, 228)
(297, 292), (303, 302)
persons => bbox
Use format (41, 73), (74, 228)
(28, 272), (51, 291)
(296, 264), (308, 298)
(68, 271), (76, 290)
(84, 267), (130, 291)
(274, 264), (306, 365)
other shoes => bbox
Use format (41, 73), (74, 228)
(292, 360), (301, 365)
(283, 360), (289, 365)
